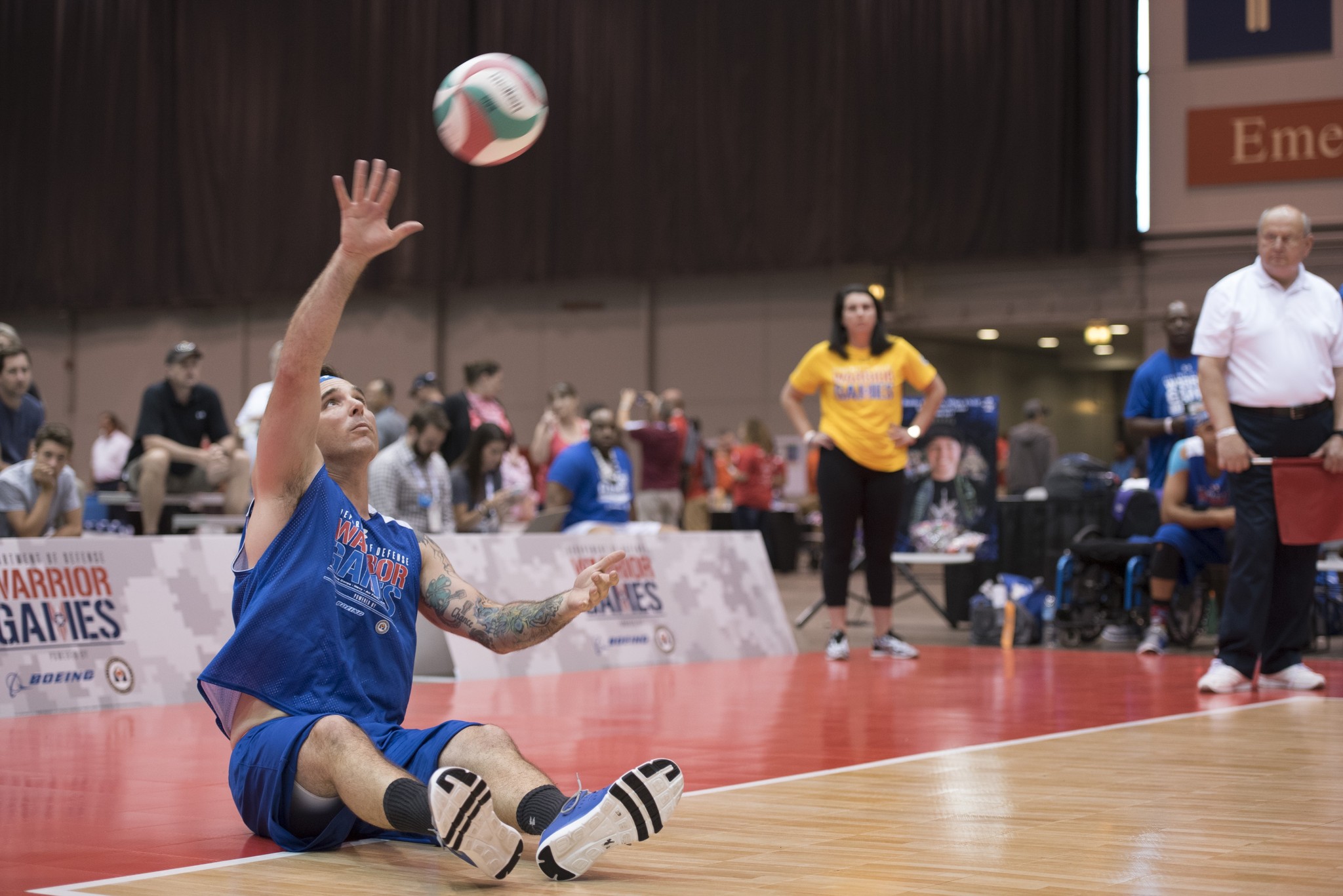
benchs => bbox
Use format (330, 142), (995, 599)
(96, 491), (249, 527)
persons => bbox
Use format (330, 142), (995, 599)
(898, 431), (995, 553)
(1008, 204), (1343, 693)
(195, 159), (684, 882)
(781, 284), (947, 661)
(0, 323), (822, 575)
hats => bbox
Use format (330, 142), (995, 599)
(164, 345), (202, 364)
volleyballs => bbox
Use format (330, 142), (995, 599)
(431, 53), (550, 169)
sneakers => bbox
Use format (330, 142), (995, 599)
(427, 766), (524, 880)
(536, 757), (684, 882)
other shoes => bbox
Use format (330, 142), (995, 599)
(1197, 662), (1252, 693)
(825, 629), (850, 659)
(870, 630), (917, 660)
(1257, 664), (1326, 690)
(1137, 628), (1167, 655)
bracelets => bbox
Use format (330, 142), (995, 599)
(804, 430), (816, 446)
(908, 425), (922, 437)
(1331, 429), (1343, 437)
(1216, 426), (1239, 439)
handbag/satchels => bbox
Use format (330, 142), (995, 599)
(971, 572), (1044, 646)
